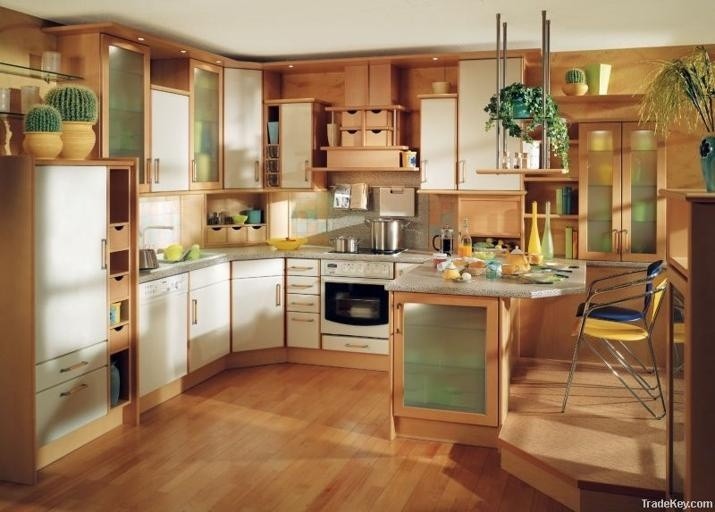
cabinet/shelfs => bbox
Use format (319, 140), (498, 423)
(0, 20), (534, 194)
(0, 157), (139, 486)
(522, 92), (667, 264)
(188, 261), (231, 375)
(392, 292), (499, 428)
(230, 257), (286, 353)
(286, 257), (320, 350)
(180, 195), (269, 247)
(139, 273), (189, 397)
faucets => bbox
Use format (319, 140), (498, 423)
(142, 225), (174, 249)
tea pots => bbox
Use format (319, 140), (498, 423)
(442, 263), (461, 281)
(507, 245), (531, 276)
(432, 223), (454, 255)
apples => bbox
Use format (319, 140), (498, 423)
(166, 244), (184, 262)
(187, 249), (200, 260)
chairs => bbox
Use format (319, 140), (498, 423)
(562, 259), (668, 420)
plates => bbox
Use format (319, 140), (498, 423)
(512, 265), (531, 275)
(545, 262), (569, 269)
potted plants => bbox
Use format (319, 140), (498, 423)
(631, 45), (715, 193)
(562, 68), (589, 96)
(483, 82), (571, 174)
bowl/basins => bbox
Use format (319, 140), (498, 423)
(468, 265), (488, 278)
(240, 208), (262, 224)
(264, 236), (307, 250)
(231, 214), (249, 225)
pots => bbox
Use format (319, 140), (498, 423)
(329, 235), (363, 254)
(364, 216), (411, 252)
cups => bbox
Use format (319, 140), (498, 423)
(267, 121), (279, 145)
(21, 84), (40, 114)
(327, 122), (341, 147)
(502, 263), (520, 275)
(1, 88), (13, 118)
(402, 153), (417, 167)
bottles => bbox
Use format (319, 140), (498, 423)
(189, 240), (200, 261)
(207, 209), (226, 225)
(459, 216), (472, 258)
(502, 150), (530, 169)
(528, 201), (544, 267)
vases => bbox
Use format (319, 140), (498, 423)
(528, 201), (541, 256)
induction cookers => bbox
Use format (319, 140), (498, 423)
(321, 246), (408, 279)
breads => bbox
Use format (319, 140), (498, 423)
(468, 261), (486, 268)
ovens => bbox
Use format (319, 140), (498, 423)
(321, 277), (389, 339)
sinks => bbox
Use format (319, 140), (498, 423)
(156, 252), (229, 264)
